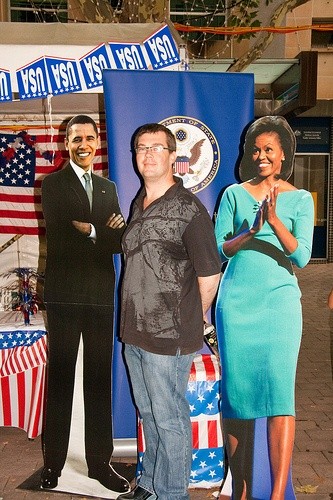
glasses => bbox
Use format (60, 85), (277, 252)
(134, 146), (169, 153)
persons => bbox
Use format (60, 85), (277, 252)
(213, 115), (314, 500)
(120, 122), (221, 500)
(40, 116), (128, 494)
(328, 288), (333, 310)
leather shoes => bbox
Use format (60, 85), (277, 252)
(87, 465), (129, 492)
(40, 465), (61, 489)
(116, 486), (152, 500)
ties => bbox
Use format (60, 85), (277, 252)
(83, 173), (93, 214)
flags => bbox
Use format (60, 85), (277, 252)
(0, 119), (109, 235)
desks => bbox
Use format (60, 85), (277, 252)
(0, 309), (225, 488)
